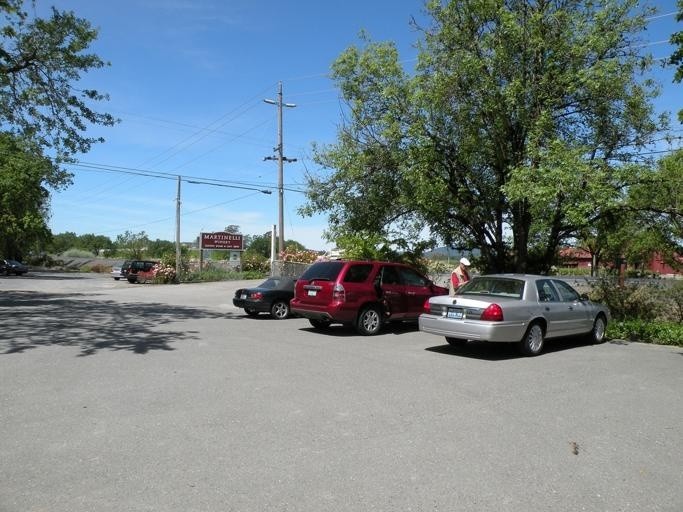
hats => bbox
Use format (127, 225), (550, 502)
(460, 257), (471, 266)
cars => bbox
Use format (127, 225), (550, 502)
(0, 258), (29, 277)
(231, 271), (301, 318)
(111, 265), (125, 280)
(417, 274), (613, 357)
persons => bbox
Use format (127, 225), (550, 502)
(448, 258), (471, 295)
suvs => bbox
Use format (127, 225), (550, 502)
(289, 258), (452, 335)
(120, 258), (161, 284)
